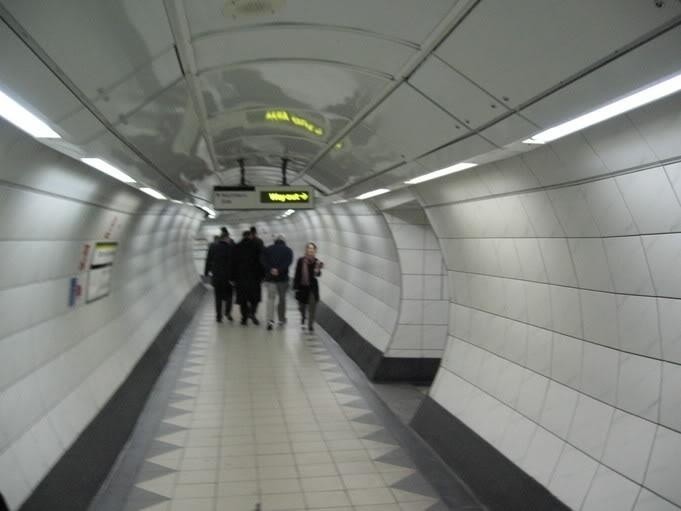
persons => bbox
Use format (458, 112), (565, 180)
(202, 226), (293, 329)
(292, 242), (324, 331)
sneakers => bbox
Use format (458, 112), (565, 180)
(215, 313), (316, 331)
